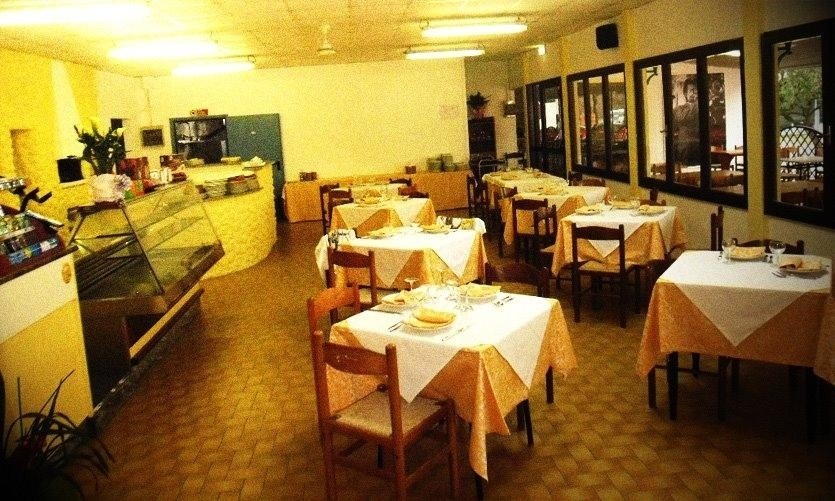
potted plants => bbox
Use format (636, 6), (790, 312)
(71, 118), (134, 205)
(467, 91), (492, 120)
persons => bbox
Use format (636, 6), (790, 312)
(672, 79), (700, 139)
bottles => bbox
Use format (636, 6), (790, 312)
(0, 212), (38, 257)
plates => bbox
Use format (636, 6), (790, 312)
(399, 310), (454, 331)
(781, 255), (825, 275)
(576, 208), (600, 215)
(639, 208), (663, 215)
(457, 284), (500, 300)
(615, 204), (633, 209)
(382, 292), (415, 307)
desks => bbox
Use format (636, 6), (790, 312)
(480, 165), (677, 327)
(635, 247), (833, 418)
(280, 167), (472, 225)
(317, 177), (441, 222)
(320, 222), (489, 287)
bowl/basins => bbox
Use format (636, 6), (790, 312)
(202, 178), (260, 198)
(722, 245), (766, 262)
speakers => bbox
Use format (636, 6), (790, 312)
(57, 155), (83, 183)
(595, 23), (619, 50)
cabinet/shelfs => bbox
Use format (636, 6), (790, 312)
(63, 184), (228, 388)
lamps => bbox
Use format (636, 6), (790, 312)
(403, 46), (487, 64)
(417, 16), (530, 43)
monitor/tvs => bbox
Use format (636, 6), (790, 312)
(169, 114), (228, 165)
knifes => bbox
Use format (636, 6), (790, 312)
(389, 320), (405, 333)
(494, 296), (513, 308)
(368, 309), (399, 315)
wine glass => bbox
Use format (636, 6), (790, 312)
(767, 239), (786, 272)
(721, 239), (738, 266)
(442, 276), (472, 311)
(404, 275), (418, 291)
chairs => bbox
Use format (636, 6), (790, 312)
(310, 324), (460, 498)
(308, 282), (360, 444)
(731, 235), (806, 393)
(326, 245), (380, 312)
(708, 206), (725, 251)
